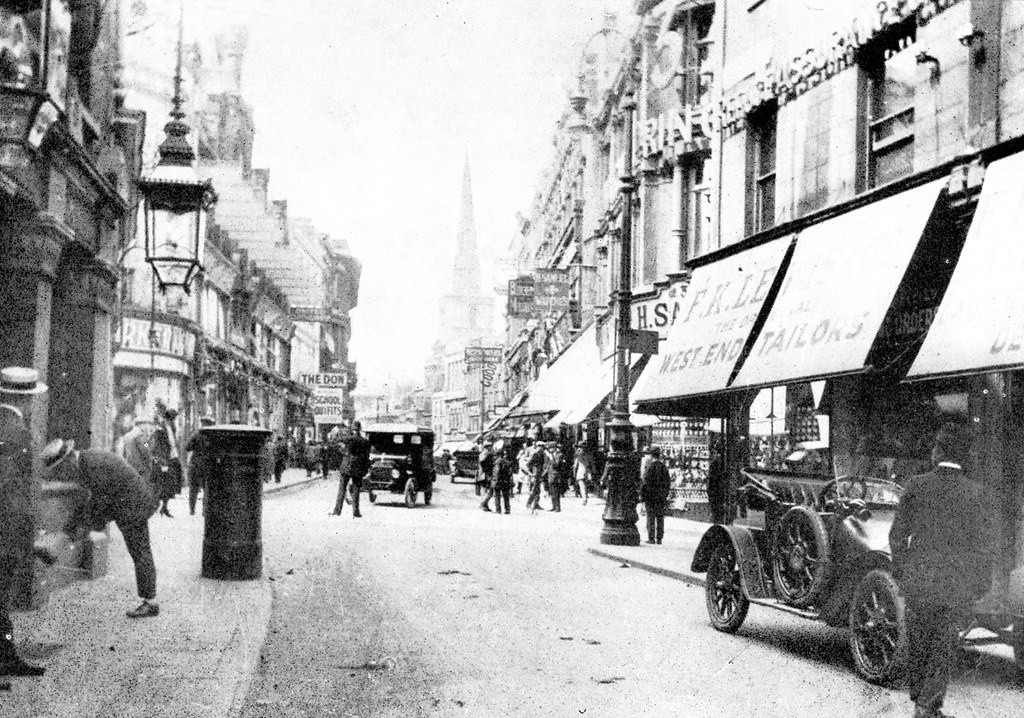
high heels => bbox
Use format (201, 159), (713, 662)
(160, 507), (172, 518)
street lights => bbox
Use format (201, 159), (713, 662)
(464, 345), (483, 496)
(569, 28), (641, 547)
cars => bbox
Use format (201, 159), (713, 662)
(450, 449), (482, 487)
(691, 468), (1024, 685)
(346, 424), (438, 509)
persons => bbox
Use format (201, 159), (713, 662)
(0, 363), (373, 678)
(889, 421), (996, 718)
(478, 432), (670, 547)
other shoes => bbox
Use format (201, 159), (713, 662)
(125, 603), (160, 618)
(533, 505), (544, 510)
(34, 546), (57, 566)
(0, 658), (44, 676)
(645, 540), (655, 544)
(526, 504), (532, 508)
(551, 509), (555, 511)
(555, 510), (560, 512)
(504, 511), (510, 514)
(657, 540), (661, 544)
(480, 503), (492, 512)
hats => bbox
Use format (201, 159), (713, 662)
(0, 366), (49, 395)
(39, 439), (74, 471)
(351, 422), (361, 431)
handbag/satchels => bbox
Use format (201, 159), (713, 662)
(478, 472), (487, 483)
